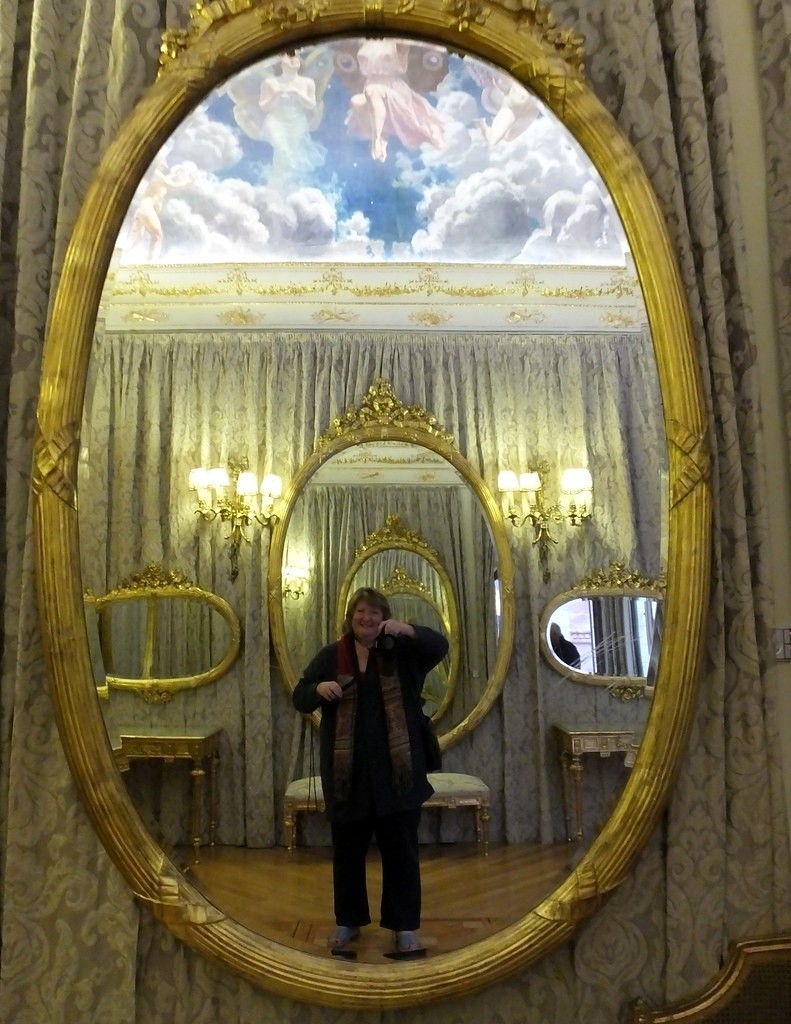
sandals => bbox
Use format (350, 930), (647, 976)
(394, 929), (420, 952)
(328, 926), (360, 948)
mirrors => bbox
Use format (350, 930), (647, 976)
(28, 0), (716, 1014)
(539, 561), (667, 703)
(375, 565), (450, 719)
(335, 513), (460, 727)
(93, 560), (243, 704)
(265, 376), (517, 758)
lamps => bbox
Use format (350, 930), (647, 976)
(188, 457), (283, 583)
(281, 540), (309, 600)
(496, 460), (592, 583)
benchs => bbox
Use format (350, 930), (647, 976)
(282, 773), (489, 859)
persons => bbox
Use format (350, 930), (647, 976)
(292, 588), (449, 953)
(550, 623), (581, 671)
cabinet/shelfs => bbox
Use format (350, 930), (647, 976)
(105, 726), (223, 865)
(551, 724), (641, 871)
